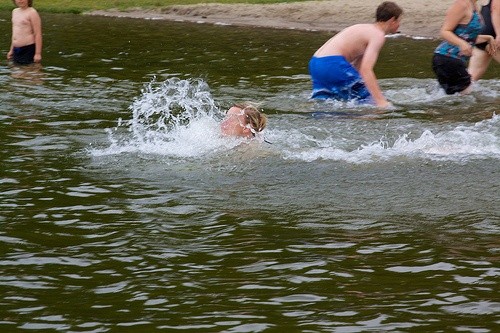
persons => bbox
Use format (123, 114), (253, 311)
(430, 0), (498, 96)
(308, 1), (403, 107)
(468, 0), (499, 85)
(6, 0), (42, 64)
(220, 102), (267, 139)
